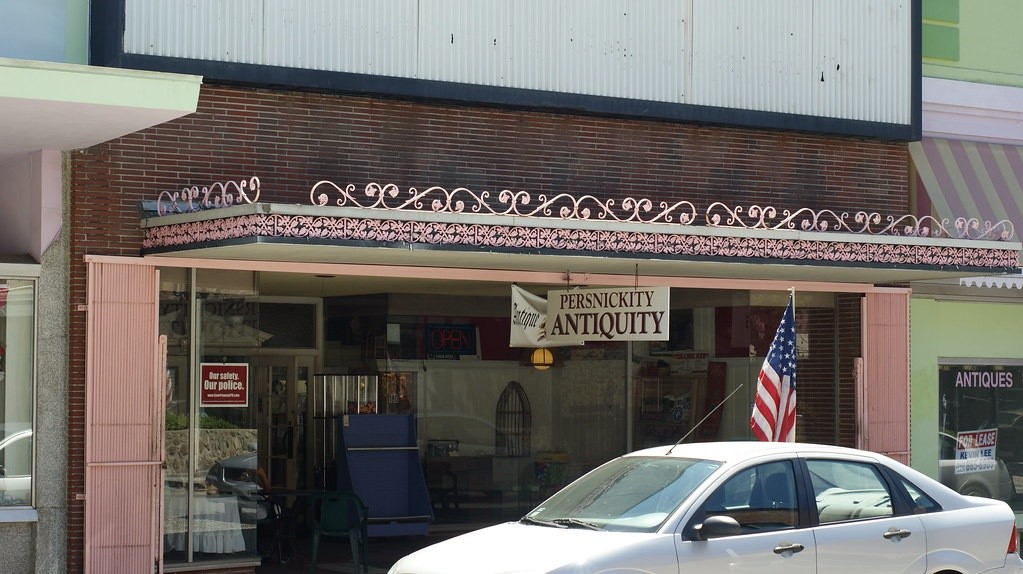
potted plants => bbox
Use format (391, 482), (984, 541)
(641, 362), (665, 377)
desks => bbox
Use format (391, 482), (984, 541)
(421, 454), (496, 520)
(163, 493), (247, 556)
(256, 488), (318, 566)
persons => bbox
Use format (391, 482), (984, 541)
(206, 449), (297, 562)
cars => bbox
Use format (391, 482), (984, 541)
(390, 440), (1023, 574)
(938, 430), (1016, 502)
(977, 408), (1023, 462)
(205, 413), (534, 525)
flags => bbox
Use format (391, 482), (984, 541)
(749, 295), (798, 442)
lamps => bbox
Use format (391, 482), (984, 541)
(518, 348), (565, 370)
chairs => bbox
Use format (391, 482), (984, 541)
(306, 491), (369, 574)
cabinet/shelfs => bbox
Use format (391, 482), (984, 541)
(633, 374), (707, 452)
